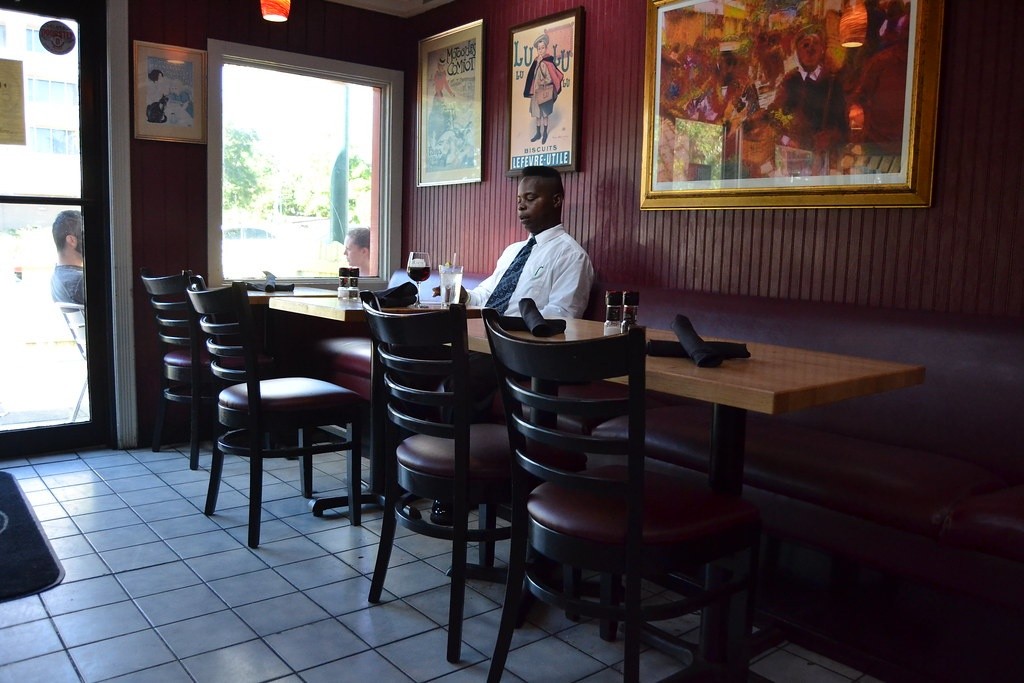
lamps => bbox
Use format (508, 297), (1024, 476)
(259, 0), (292, 22)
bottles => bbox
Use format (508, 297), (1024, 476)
(604, 291), (622, 336)
(621, 291), (639, 334)
(338, 268), (349, 300)
(349, 268), (359, 300)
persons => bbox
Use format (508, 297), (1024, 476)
(51, 211), (84, 311)
(433, 167), (595, 319)
(344, 228), (370, 275)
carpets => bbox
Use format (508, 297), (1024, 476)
(0, 470), (66, 608)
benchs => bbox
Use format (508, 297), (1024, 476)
(318, 264), (1024, 683)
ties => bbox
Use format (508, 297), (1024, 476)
(484, 236), (537, 317)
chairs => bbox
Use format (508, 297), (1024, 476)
(55, 301), (88, 423)
(484, 305), (763, 683)
(358, 299), (582, 665)
(185, 274), (362, 551)
(138, 265), (279, 473)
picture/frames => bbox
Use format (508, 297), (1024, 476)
(639, 0), (946, 210)
(416, 17), (486, 187)
(133, 39), (208, 145)
(504, 6), (585, 177)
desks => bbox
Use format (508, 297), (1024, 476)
(264, 295), (494, 524)
(443, 313), (927, 683)
(245, 285), (338, 464)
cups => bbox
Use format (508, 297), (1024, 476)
(439, 265), (464, 309)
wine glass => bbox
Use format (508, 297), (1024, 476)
(407, 252), (431, 309)
